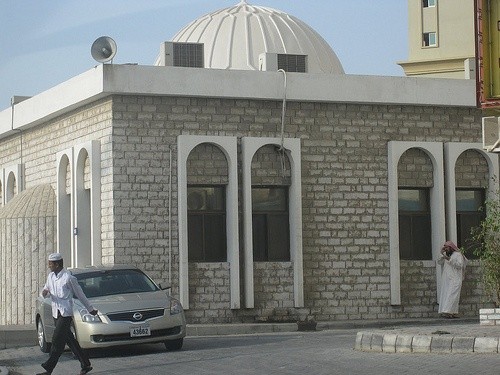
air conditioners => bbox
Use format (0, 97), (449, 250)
(160, 41), (205, 68)
(258, 52), (309, 73)
(187, 189), (207, 211)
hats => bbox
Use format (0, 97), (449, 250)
(48, 253), (64, 261)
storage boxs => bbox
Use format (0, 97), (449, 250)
(399, 189), (427, 212)
(457, 190), (484, 213)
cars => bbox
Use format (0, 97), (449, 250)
(35, 264), (187, 357)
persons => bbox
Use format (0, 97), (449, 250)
(437, 241), (464, 319)
(35, 253), (98, 375)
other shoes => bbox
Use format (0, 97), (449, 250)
(41, 362), (53, 375)
(80, 366), (92, 374)
(441, 315), (459, 319)
